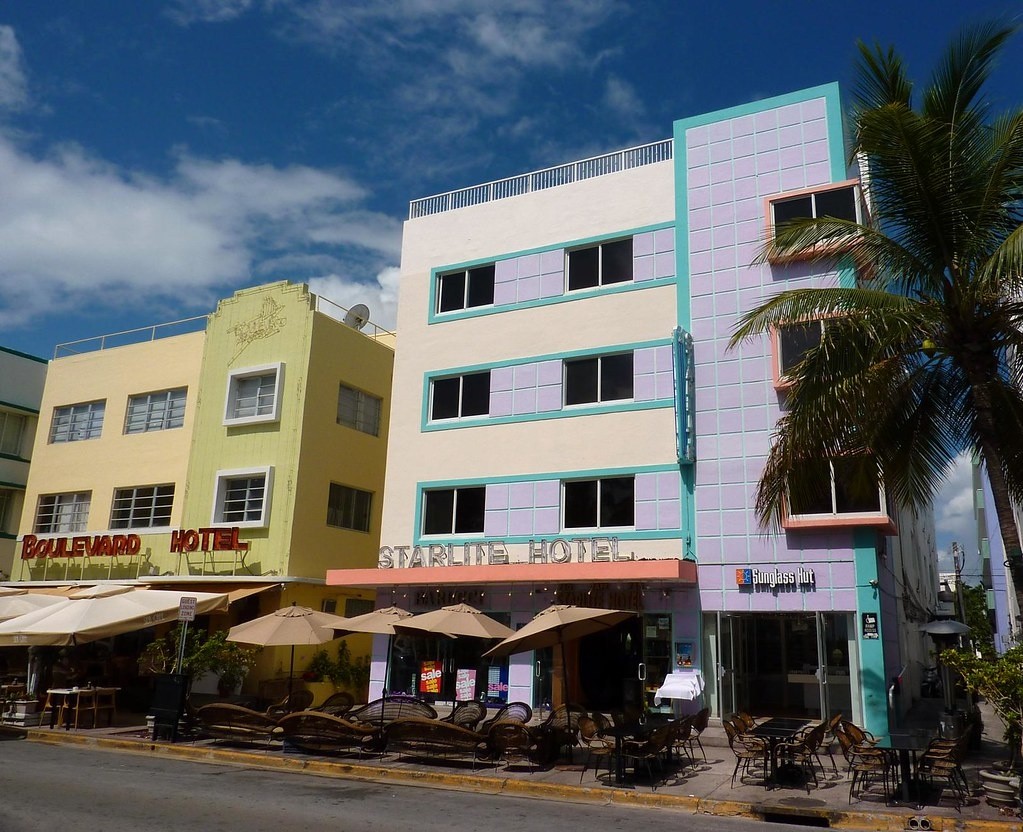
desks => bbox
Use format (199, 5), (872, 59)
(873, 733), (926, 811)
(743, 718), (812, 791)
(38, 687), (121, 730)
(598, 713), (671, 791)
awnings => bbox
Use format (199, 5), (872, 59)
(140, 583), (278, 614)
(0, 583), (229, 647)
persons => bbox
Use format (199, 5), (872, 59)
(393, 635), (498, 702)
(30, 646), (62, 694)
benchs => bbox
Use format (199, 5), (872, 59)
(191, 695), (484, 771)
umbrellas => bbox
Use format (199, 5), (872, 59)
(391, 602), (517, 725)
(321, 602), (459, 735)
(480, 601), (639, 761)
(226, 602), (357, 712)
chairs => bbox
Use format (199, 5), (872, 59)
(722, 709), (970, 815)
(439, 700), (709, 791)
(265, 689), (314, 720)
(310, 690), (355, 718)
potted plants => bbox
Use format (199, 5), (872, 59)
(5, 691), (40, 713)
(217, 644), (265, 695)
